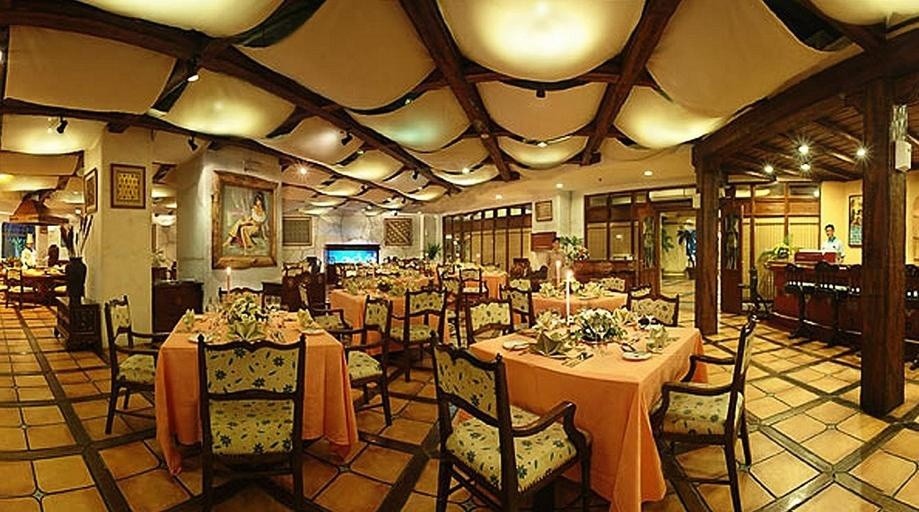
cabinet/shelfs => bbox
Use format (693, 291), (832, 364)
(53, 296), (102, 355)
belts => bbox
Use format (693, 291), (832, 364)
(586, 315), (609, 355)
(264, 295), (281, 310)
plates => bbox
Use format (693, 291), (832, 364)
(53, 296), (102, 355)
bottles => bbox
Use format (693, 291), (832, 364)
(26, 234), (33, 243)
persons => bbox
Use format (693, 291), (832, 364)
(20, 243), (37, 272)
(546, 238), (565, 282)
(820, 224), (841, 258)
(224, 191), (268, 254)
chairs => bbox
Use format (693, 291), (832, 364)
(650, 314), (758, 512)
(105, 295), (173, 435)
(780, 262), (918, 372)
(217, 259), (682, 345)
(429, 328), (593, 511)
(1, 260), (68, 310)
(324, 293), (395, 423)
(197, 333), (307, 512)
(381, 288), (450, 382)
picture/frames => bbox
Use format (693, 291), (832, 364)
(82, 167), (99, 214)
(110, 163), (147, 209)
(283, 216), (315, 246)
(534, 200), (553, 222)
(209, 169), (278, 270)
(383, 217), (413, 246)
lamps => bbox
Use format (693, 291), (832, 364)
(780, 262), (918, 372)
(184, 56), (203, 83)
(341, 127), (354, 146)
(412, 166), (420, 179)
(1, 260), (68, 310)
(187, 135), (199, 152)
(55, 116), (68, 135)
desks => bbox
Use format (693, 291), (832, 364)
(156, 312), (343, 468)
(468, 314), (700, 511)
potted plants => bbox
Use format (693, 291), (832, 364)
(110, 163), (147, 209)
(534, 200), (553, 222)
(82, 167), (99, 214)
(283, 216), (315, 246)
(383, 217), (413, 246)
(209, 169), (278, 270)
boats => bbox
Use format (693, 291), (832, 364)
(895, 140), (912, 172)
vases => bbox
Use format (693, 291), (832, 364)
(63, 257), (87, 303)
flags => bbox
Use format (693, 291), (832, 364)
(63, 257), (87, 303)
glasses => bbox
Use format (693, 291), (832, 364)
(55, 116), (68, 135)
(184, 56), (203, 83)
(187, 135), (199, 152)
(412, 166), (420, 179)
(341, 127), (354, 146)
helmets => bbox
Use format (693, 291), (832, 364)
(503, 339), (531, 351)
(622, 349), (650, 361)
(187, 308), (324, 344)
